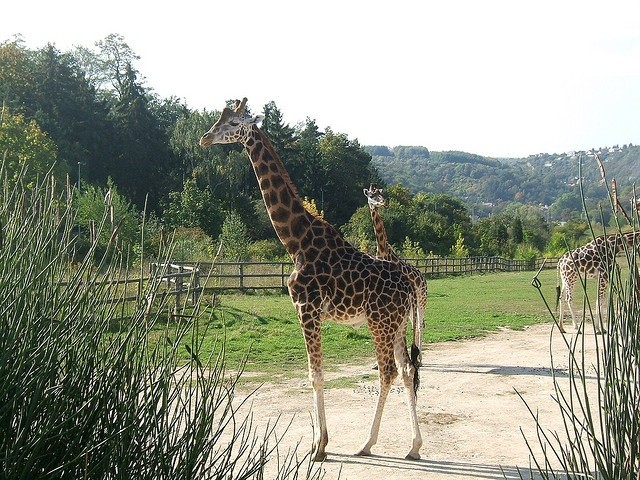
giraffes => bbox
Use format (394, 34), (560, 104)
(363, 182), (428, 370)
(198, 97), (423, 463)
(556, 230), (640, 335)
